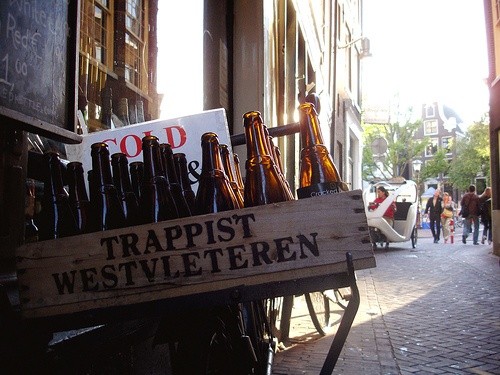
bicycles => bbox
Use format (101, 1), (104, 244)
(144, 95), (320, 375)
(306, 287), (351, 335)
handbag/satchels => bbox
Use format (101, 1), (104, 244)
(442, 208), (454, 217)
(463, 205), (468, 217)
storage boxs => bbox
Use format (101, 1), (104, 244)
(13, 187), (376, 336)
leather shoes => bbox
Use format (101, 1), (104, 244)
(474, 241), (478, 245)
(463, 235), (466, 243)
(435, 238), (440, 242)
(434, 235), (437, 242)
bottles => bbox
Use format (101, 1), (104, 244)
(135, 136), (195, 227)
(84, 142), (144, 235)
(18, 150), (93, 248)
(298, 102), (343, 199)
(242, 110), (295, 209)
(192, 132), (244, 218)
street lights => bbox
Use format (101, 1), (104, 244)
(413, 160), (423, 226)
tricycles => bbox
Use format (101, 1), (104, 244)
(362, 177), (418, 250)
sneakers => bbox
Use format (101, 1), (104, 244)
(481, 237), (487, 244)
(488, 240), (491, 243)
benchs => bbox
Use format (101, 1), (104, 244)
(367, 200), (411, 221)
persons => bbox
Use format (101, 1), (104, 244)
(448, 196), (454, 205)
(424, 188), (445, 244)
(481, 185), (492, 244)
(463, 184), (484, 246)
(372, 186), (396, 228)
(442, 191), (455, 243)
